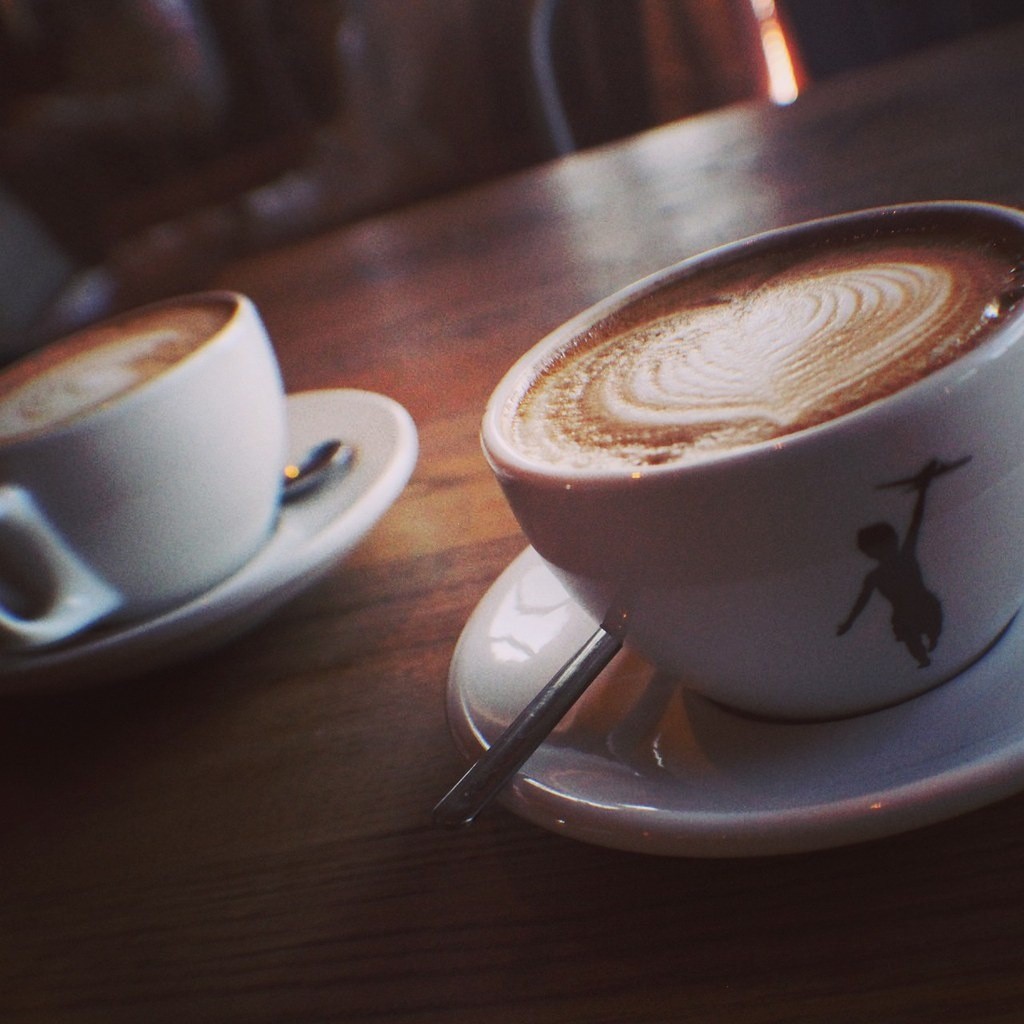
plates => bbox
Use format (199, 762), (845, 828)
(445, 537), (1024, 859)
(0, 387), (420, 691)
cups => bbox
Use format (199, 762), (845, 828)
(0, 290), (289, 648)
(478, 199), (1024, 724)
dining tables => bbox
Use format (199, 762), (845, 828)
(0, 23), (1024, 1024)
